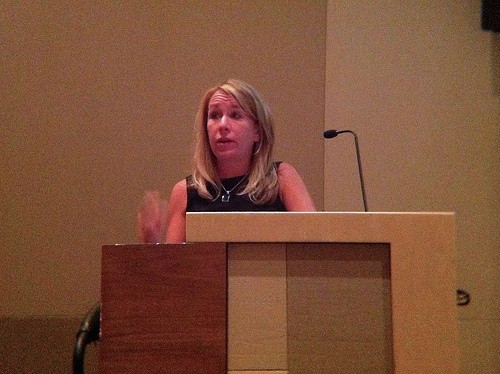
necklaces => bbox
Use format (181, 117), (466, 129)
(219, 173), (248, 202)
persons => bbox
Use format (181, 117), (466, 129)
(137, 79), (318, 242)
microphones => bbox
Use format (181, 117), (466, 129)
(323, 129), (368, 211)
